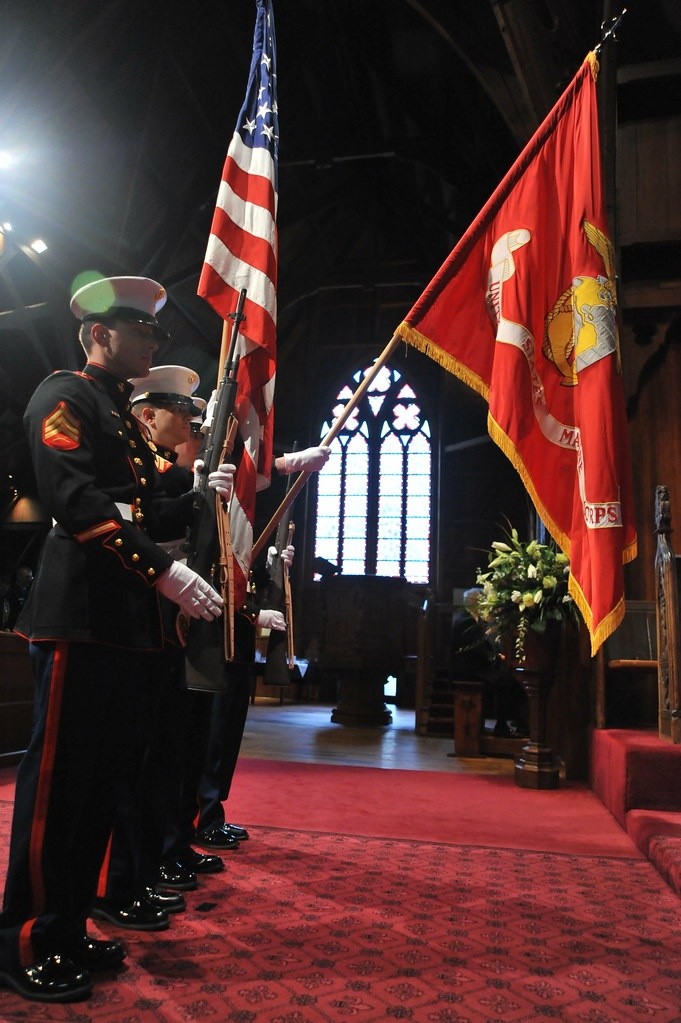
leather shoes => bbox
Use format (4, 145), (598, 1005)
(87, 895), (170, 931)
(133, 881), (185, 914)
(151, 861), (197, 891)
(53, 934), (126, 975)
(220, 822), (249, 840)
(179, 848), (225, 874)
(0, 932), (93, 1002)
(189, 828), (240, 849)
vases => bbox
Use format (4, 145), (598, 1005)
(498, 619), (585, 791)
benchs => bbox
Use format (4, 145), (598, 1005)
(588, 728), (681, 830)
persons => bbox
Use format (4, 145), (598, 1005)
(12, 276), (330, 1007)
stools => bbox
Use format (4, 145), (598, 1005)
(447, 681), (488, 758)
(251, 662), (286, 706)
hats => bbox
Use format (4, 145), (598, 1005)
(190, 396), (207, 431)
(126, 365), (202, 418)
(70, 276), (170, 341)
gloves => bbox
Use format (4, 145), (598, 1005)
(266, 546), (295, 576)
(284, 446), (332, 474)
(151, 560), (224, 622)
(253, 609), (287, 631)
(193, 460), (236, 503)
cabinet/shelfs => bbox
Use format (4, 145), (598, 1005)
(0, 632), (36, 770)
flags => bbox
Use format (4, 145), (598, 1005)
(394, 52), (638, 660)
(196, 2), (278, 614)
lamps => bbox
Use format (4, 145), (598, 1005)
(0, 491), (48, 632)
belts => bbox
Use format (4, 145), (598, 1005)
(246, 581), (256, 594)
(156, 537), (191, 559)
(52, 502), (135, 528)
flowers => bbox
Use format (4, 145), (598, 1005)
(435, 512), (581, 663)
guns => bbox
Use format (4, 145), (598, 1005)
(259, 436), (299, 687)
(176, 288), (252, 668)
